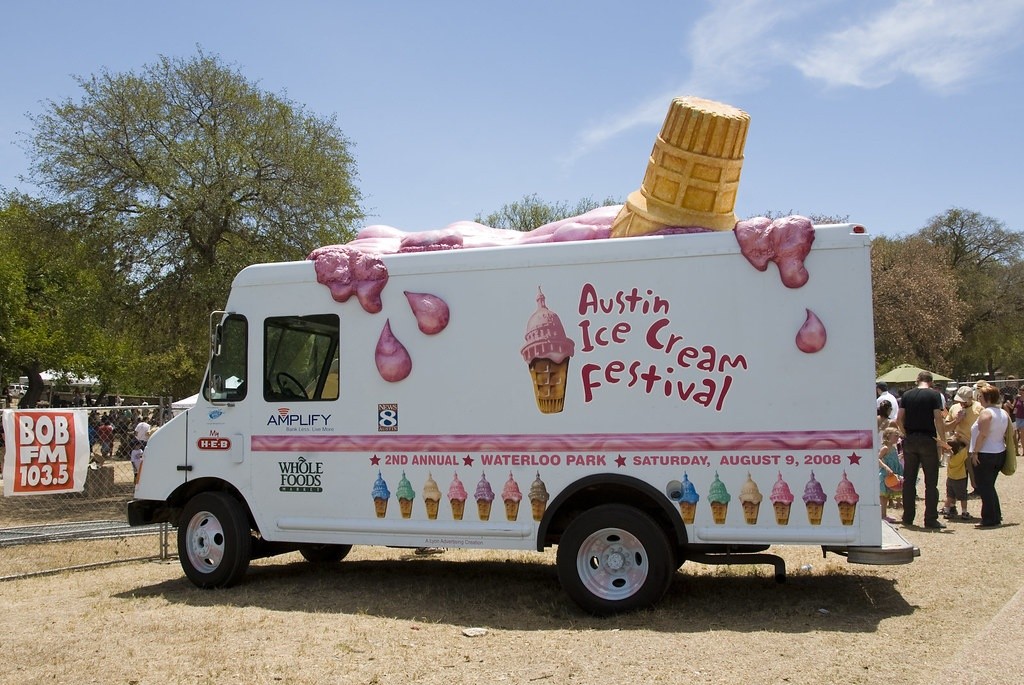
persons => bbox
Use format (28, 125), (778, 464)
(2, 384), (14, 408)
(0, 418), (7, 479)
(88, 399), (175, 481)
(876, 371), (1024, 529)
(46, 388), (92, 406)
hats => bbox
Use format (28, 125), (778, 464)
(973, 380), (990, 390)
(876, 382), (887, 388)
(954, 386), (973, 402)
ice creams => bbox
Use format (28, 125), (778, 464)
(304, 96), (750, 285)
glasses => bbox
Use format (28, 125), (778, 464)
(1019, 389), (1024, 391)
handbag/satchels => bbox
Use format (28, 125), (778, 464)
(1000, 409), (1017, 475)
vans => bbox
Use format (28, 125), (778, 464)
(126, 223), (921, 617)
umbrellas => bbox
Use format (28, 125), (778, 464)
(875, 363), (954, 388)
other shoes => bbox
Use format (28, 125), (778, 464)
(883, 516), (896, 523)
(962, 513), (974, 520)
(897, 518), (914, 525)
(925, 521), (946, 528)
(943, 511), (950, 519)
(937, 506), (958, 515)
(974, 522), (996, 529)
